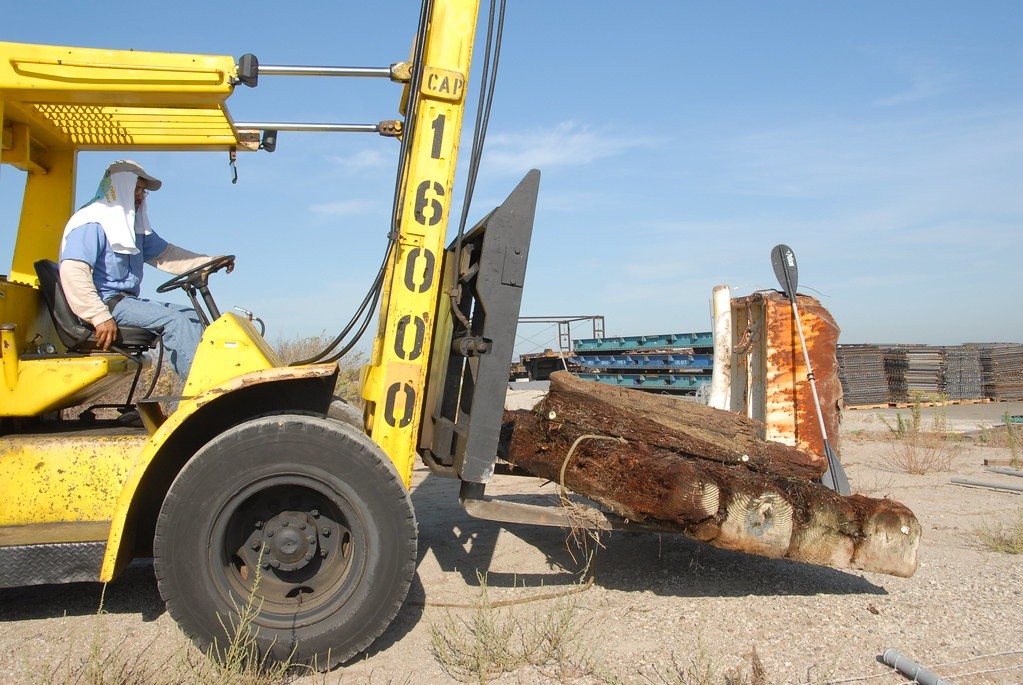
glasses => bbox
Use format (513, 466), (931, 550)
(133, 185), (149, 198)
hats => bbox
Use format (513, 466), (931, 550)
(105, 160), (162, 192)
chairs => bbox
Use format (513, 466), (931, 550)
(34, 258), (164, 352)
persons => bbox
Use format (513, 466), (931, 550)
(57, 160), (235, 380)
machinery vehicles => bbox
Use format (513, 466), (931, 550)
(0, 0), (924, 682)
